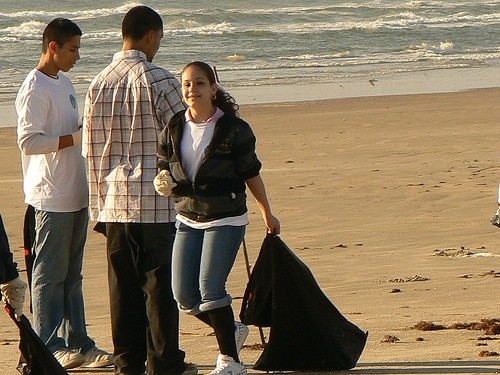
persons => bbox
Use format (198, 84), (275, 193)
(15, 17), (114, 368)
(81, 6), (197, 375)
(153, 61), (280, 375)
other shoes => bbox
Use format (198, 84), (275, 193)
(181, 364), (198, 375)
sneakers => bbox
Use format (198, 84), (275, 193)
(217, 321), (249, 366)
(80, 347), (114, 367)
(53, 348), (85, 370)
(206, 355), (247, 375)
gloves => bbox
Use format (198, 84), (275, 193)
(1, 277), (28, 321)
(153, 169), (177, 197)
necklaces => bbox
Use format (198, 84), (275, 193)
(38, 68), (59, 80)
